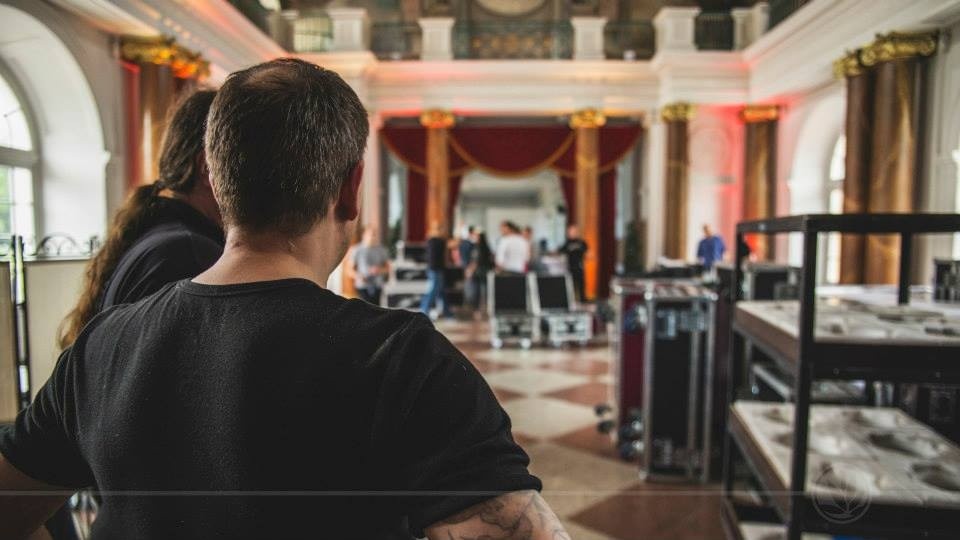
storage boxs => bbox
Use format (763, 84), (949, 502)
(608, 261), (798, 486)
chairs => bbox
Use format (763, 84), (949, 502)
(378, 238), (595, 350)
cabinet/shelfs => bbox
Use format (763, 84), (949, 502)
(718, 213), (960, 540)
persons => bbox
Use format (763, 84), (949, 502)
(1, 57), (572, 539)
(418, 220), (596, 321)
(696, 223), (726, 271)
(348, 225), (392, 308)
